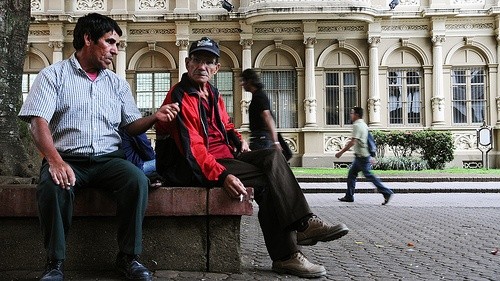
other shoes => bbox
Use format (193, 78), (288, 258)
(338, 194), (354, 202)
(382, 190), (394, 206)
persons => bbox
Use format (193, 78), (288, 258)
(154, 37), (348, 279)
(335, 107), (394, 206)
(240, 67), (283, 152)
(120, 131), (164, 186)
(17, 12), (180, 281)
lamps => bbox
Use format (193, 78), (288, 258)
(222, 0), (234, 12)
(389, 0), (399, 9)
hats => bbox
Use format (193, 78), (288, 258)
(189, 36), (220, 58)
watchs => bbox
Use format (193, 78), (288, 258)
(272, 142), (280, 146)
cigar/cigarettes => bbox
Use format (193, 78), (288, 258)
(238, 193), (245, 202)
(66, 178), (77, 186)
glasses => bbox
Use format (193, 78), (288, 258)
(190, 58), (217, 67)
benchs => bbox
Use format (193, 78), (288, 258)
(0, 183), (255, 272)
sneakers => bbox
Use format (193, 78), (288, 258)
(40, 259), (66, 281)
(296, 214), (349, 246)
(115, 252), (152, 281)
(272, 250), (326, 278)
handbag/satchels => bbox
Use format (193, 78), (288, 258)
(368, 132), (376, 157)
